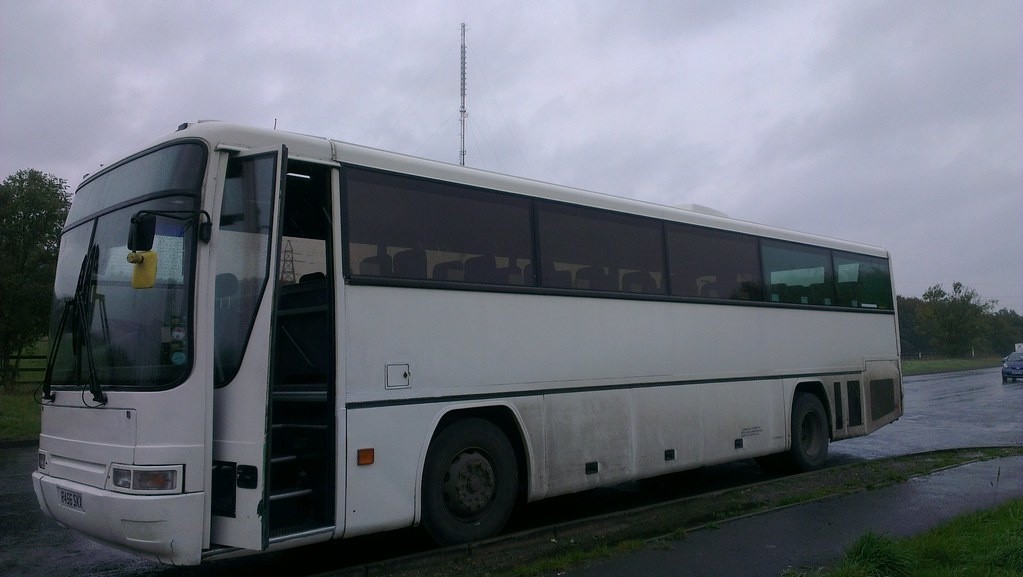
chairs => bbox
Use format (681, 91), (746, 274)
(213, 246), (871, 382)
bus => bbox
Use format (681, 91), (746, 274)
(31, 117), (907, 568)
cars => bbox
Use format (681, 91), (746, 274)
(1001, 352), (1023, 380)
